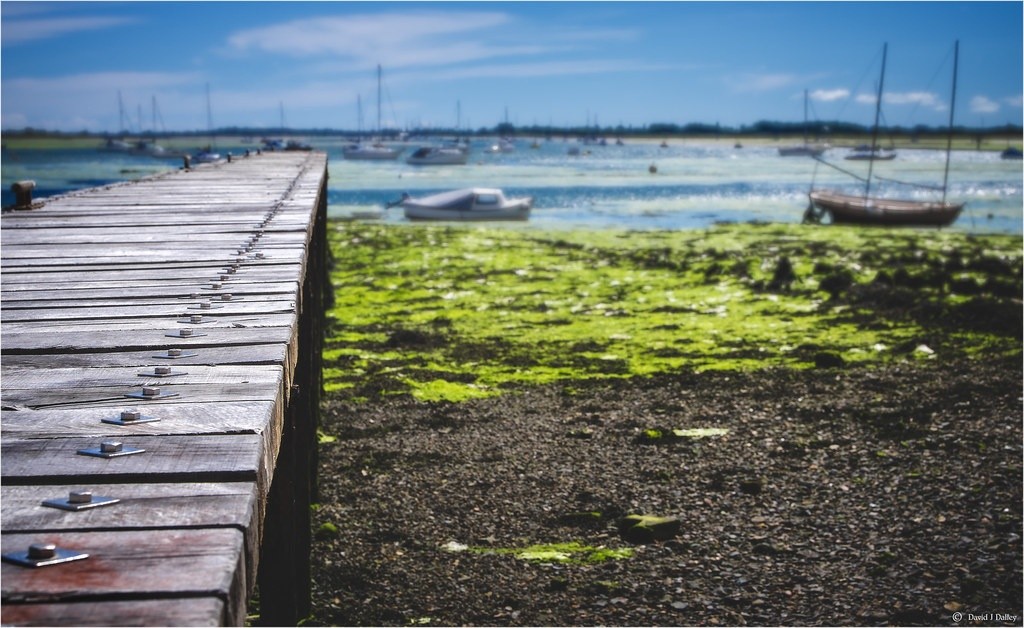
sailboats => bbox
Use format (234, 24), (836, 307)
(102, 60), (595, 165)
(806, 37), (972, 231)
(777, 87), (835, 158)
(842, 80), (898, 162)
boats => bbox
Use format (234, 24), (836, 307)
(386, 186), (534, 222)
